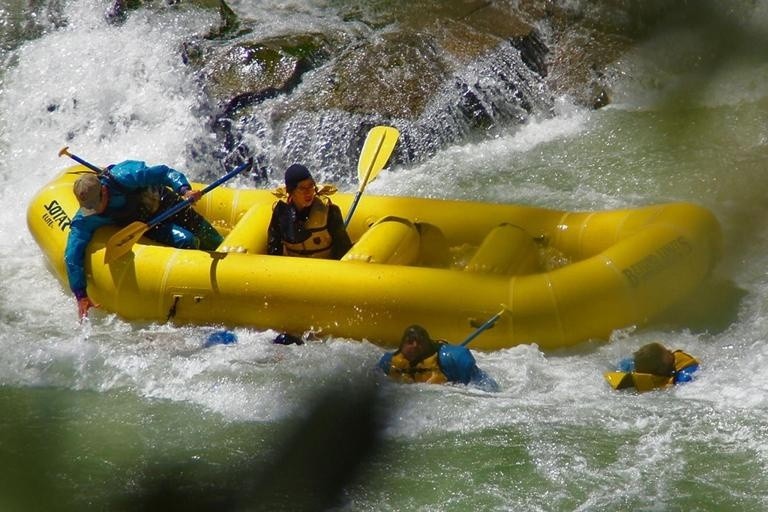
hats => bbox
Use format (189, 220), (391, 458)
(285, 164), (312, 192)
(74, 174), (102, 217)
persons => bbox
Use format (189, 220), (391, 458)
(266, 163), (353, 259)
(63, 160), (223, 323)
(605, 340), (698, 393)
(377, 324), (501, 392)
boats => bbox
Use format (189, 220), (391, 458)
(21, 160), (723, 352)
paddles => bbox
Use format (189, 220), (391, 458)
(344, 126), (400, 229)
(104, 164), (247, 264)
